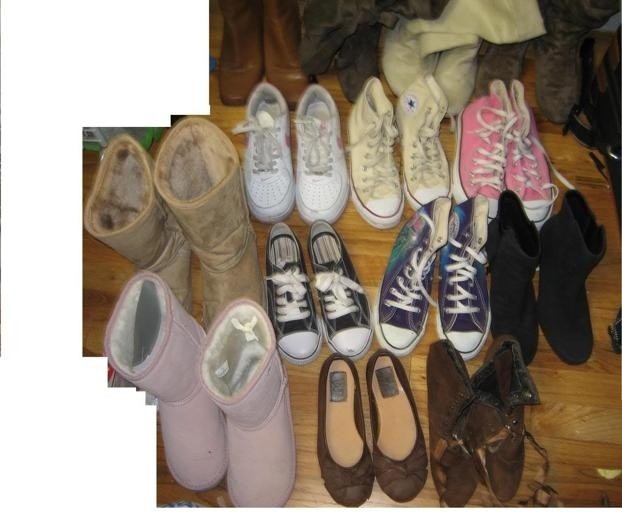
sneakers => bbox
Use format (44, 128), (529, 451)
(264, 223), (320, 365)
(231, 83), (293, 224)
(319, 335), (556, 501)
(305, 220), (374, 360)
(298, 85), (351, 228)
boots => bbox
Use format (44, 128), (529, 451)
(396, 75), (453, 213)
(83, 135), (193, 315)
(370, 196), (452, 356)
(199, 299), (295, 508)
(220, 0), (622, 122)
(153, 117), (270, 335)
(105, 271), (229, 490)
(346, 77), (404, 231)
(451, 79), (516, 224)
(485, 190), (539, 365)
(539, 191), (605, 366)
(437, 194), (490, 363)
(505, 81), (576, 229)
